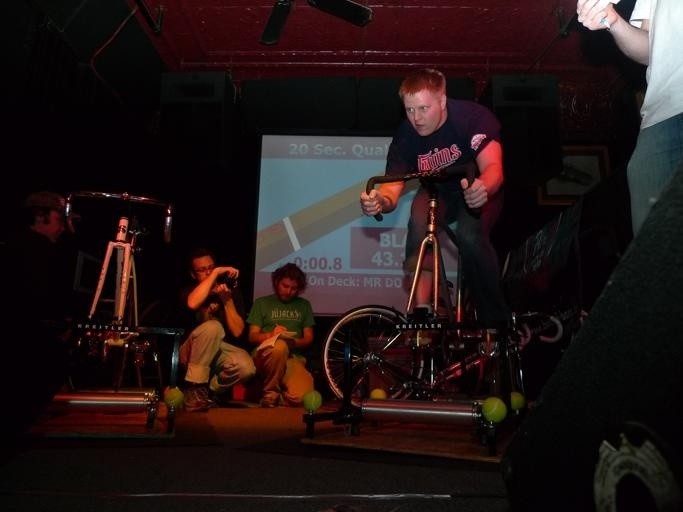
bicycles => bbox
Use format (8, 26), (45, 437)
(319, 171), (527, 411)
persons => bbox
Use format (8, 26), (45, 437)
(244, 262), (317, 408)
(574, 1), (682, 237)
(21, 188), (68, 245)
(174, 248), (258, 414)
(359, 64), (509, 332)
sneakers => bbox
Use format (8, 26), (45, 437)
(196, 385), (216, 409)
(257, 390), (280, 409)
(183, 387), (209, 412)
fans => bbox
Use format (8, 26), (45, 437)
(260, 0), (374, 46)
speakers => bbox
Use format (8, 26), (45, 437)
(497, 159), (683, 512)
(157, 70), (562, 140)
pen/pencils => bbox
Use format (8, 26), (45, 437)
(275, 323), (279, 327)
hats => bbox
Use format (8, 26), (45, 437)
(24, 191), (82, 220)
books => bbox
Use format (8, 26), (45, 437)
(254, 330), (297, 353)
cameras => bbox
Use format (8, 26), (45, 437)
(217, 273), (240, 289)
